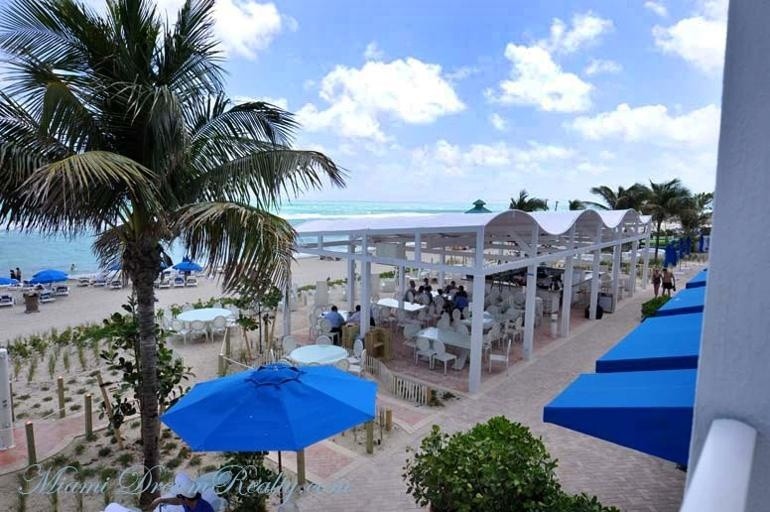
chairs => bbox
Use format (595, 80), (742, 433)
(162, 304), (238, 344)
(282, 290), (545, 380)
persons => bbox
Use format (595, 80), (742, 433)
(146, 481), (215, 512)
(16, 266), (22, 281)
(653, 268), (663, 297)
(662, 268), (676, 298)
(370, 307), (375, 326)
(71, 264), (76, 270)
(325, 305), (344, 346)
(35, 284), (43, 289)
(519, 272), (564, 306)
(405, 277), (468, 321)
(10, 269), (15, 279)
(349, 305), (360, 324)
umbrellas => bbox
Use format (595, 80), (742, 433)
(0, 277), (17, 286)
(173, 261), (202, 286)
(664, 233), (704, 271)
(31, 269), (68, 290)
(159, 362), (377, 504)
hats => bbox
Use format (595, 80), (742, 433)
(171, 473), (199, 498)
